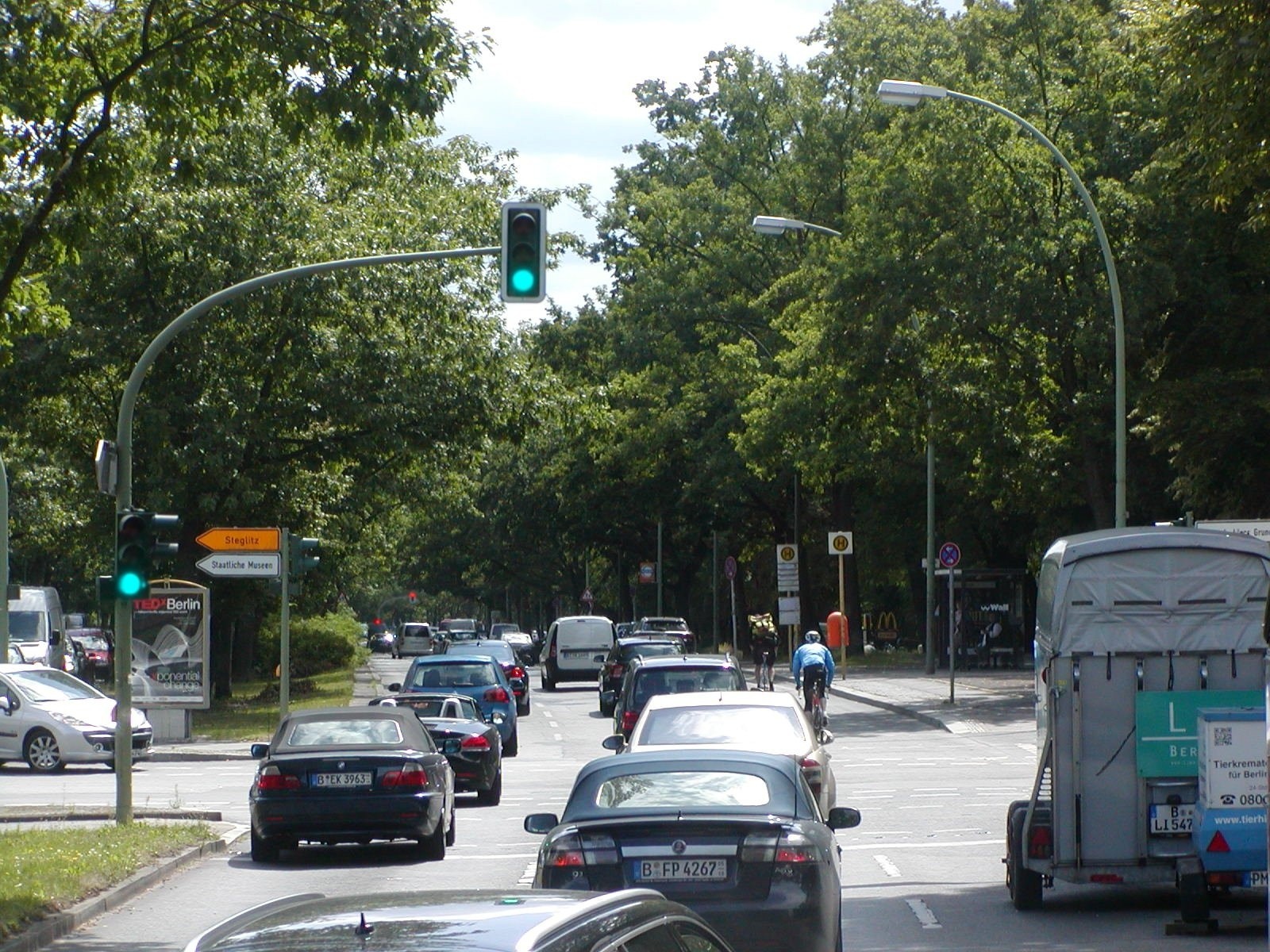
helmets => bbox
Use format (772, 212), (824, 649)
(805, 631), (821, 643)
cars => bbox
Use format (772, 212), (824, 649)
(388, 654), (526, 758)
(0, 662), (155, 774)
(361, 616), (698, 716)
(65, 612), (116, 680)
(250, 704), (461, 863)
(367, 692), (509, 806)
(601, 690), (836, 824)
(523, 748), (862, 952)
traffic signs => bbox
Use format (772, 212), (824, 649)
(195, 527), (282, 552)
(94, 439), (117, 496)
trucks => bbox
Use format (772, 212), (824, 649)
(1000, 511), (1270, 938)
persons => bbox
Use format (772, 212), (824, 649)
(792, 630), (836, 726)
(954, 600), (961, 670)
(863, 641), (925, 656)
(749, 613), (778, 692)
(974, 613), (1003, 670)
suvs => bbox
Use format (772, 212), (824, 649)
(181, 886), (738, 952)
(594, 634), (688, 718)
(599, 651), (764, 743)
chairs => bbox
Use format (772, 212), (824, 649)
(701, 672), (727, 691)
(640, 672), (668, 694)
(421, 670), (442, 686)
(470, 672), (485, 686)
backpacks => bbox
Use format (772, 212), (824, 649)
(749, 613), (774, 637)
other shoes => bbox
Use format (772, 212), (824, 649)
(823, 715), (828, 727)
(769, 680), (774, 691)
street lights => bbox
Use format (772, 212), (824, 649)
(874, 76), (1126, 527)
(95, 575), (113, 627)
(752, 215), (935, 677)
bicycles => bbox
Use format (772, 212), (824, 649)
(757, 643), (782, 692)
(796, 677), (831, 736)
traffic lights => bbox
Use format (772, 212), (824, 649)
(290, 535), (321, 574)
(195, 552), (281, 578)
(116, 511), (150, 599)
(149, 513), (180, 558)
(502, 200), (546, 303)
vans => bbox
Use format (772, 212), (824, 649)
(6, 585), (65, 671)
